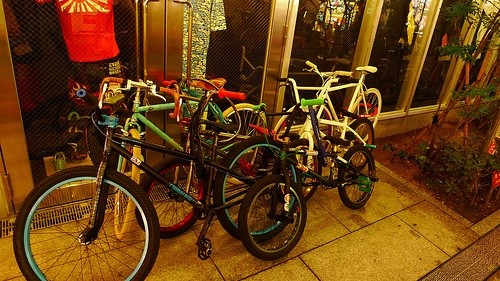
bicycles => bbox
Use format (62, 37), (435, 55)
(12, 60), (383, 281)
